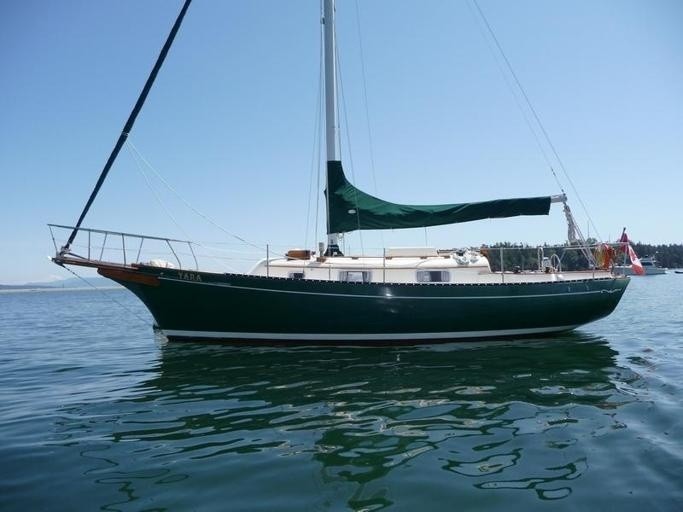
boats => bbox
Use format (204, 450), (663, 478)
(612, 255), (667, 275)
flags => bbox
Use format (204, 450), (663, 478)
(620, 233), (646, 276)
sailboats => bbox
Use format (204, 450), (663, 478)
(43, 0), (644, 349)
